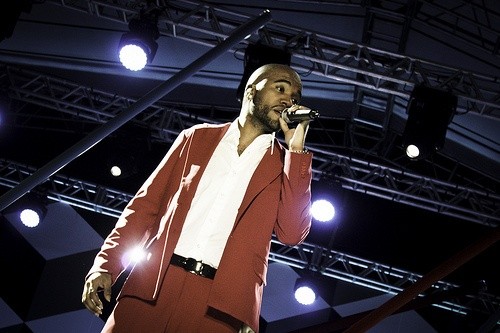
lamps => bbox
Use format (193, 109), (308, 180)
(234, 37), (292, 107)
(293, 270), (326, 305)
(310, 177), (343, 222)
(118, 9), (161, 73)
(401, 83), (455, 161)
(0, 0), (45, 45)
(20, 195), (48, 227)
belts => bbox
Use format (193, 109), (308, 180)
(169, 253), (217, 279)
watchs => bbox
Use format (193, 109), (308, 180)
(289, 146), (309, 155)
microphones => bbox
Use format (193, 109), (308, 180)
(281, 107), (320, 123)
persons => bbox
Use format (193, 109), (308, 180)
(82, 64), (310, 333)
